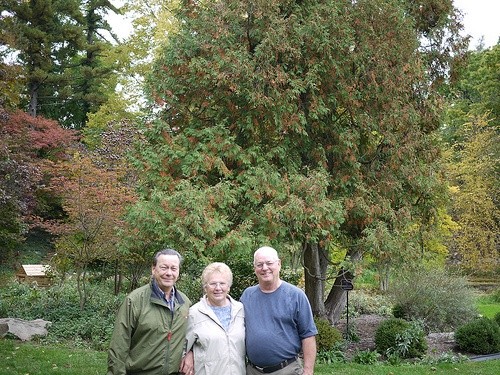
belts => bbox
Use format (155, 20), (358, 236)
(248, 357), (296, 374)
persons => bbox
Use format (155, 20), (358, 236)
(238, 246), (318, 375)
(182, 262), (246, 375)
(108, 249), (194, 375)
